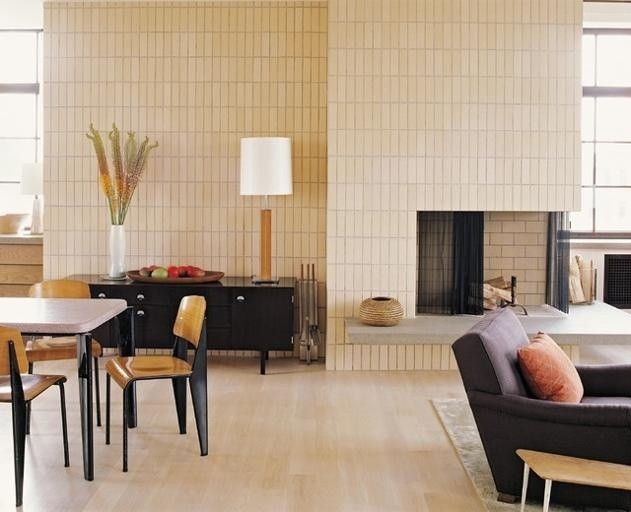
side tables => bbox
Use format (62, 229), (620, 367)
(516, 449), (631, 512)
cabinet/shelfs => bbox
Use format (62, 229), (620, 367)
(0, 238), (43, 296)
(52, 274), (296, 375)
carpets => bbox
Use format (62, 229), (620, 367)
(431, 397), (580, 512)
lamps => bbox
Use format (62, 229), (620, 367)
(21, 161), (43, 234)
(240, 137), (293, 284)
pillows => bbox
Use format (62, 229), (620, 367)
(517, 329), (584, 402)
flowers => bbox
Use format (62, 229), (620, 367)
(86, 123), (158, 224)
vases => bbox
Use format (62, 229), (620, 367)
(110, 225), (125, 278)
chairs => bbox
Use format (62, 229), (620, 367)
(105, 296), (208, 472)
(1, 324), (69, 507)
(25, 280), (102, 435)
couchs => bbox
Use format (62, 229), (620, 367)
(451, 307), (631, 511)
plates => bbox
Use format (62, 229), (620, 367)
(126, 269), (226, 284)
